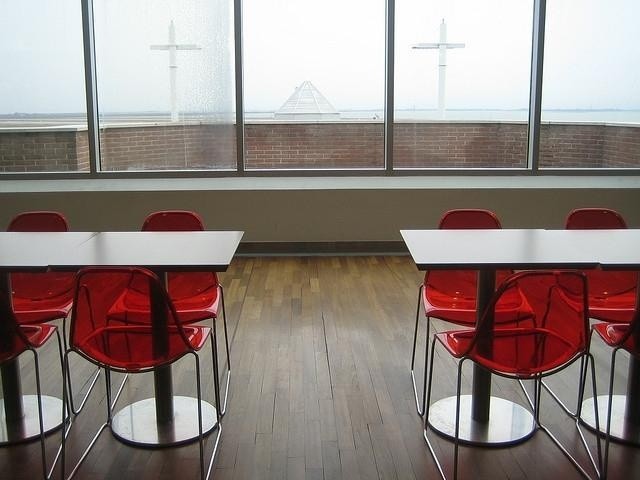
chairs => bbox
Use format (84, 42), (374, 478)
(106, 209), (232, 419)
(1, 264), (74, 480)
(58, 266), (223, 480)
(420, 268), (604, 479)
(534, 206), (639, 418)
(409, 208), (538, 415)
(575, 309), (639, 478)
(2, 212), (106, 419)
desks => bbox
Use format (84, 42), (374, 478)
(0, 230), (246, 449)
(398, 228), (640, 449)
